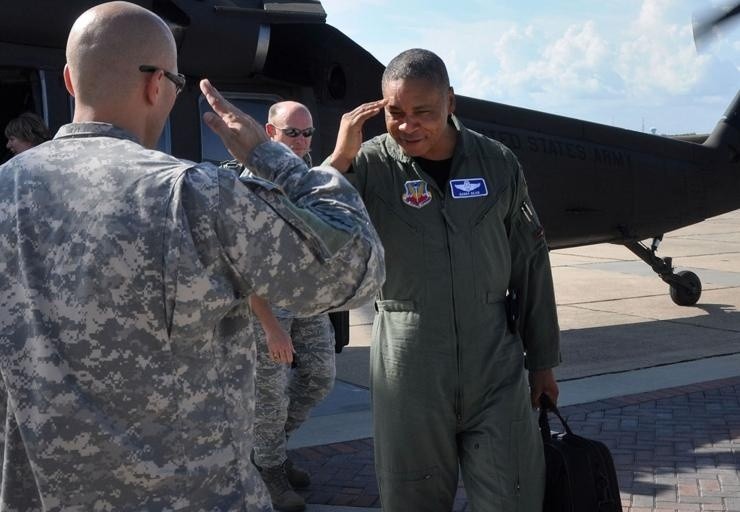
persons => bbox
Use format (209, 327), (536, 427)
(226, 100), (337, 512)
(318, 49), (562, 512)
(0, 0), (389, 512)
(6, 111), (49, 156)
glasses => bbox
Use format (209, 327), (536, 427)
(139, 64), (186, 95)
(272, 125), (316, 138)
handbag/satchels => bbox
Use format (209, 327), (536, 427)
(540, 394), (623, 512)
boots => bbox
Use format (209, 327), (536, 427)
(250, 449), (311, 512)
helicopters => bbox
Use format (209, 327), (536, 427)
(0, 0), (740, 307)
